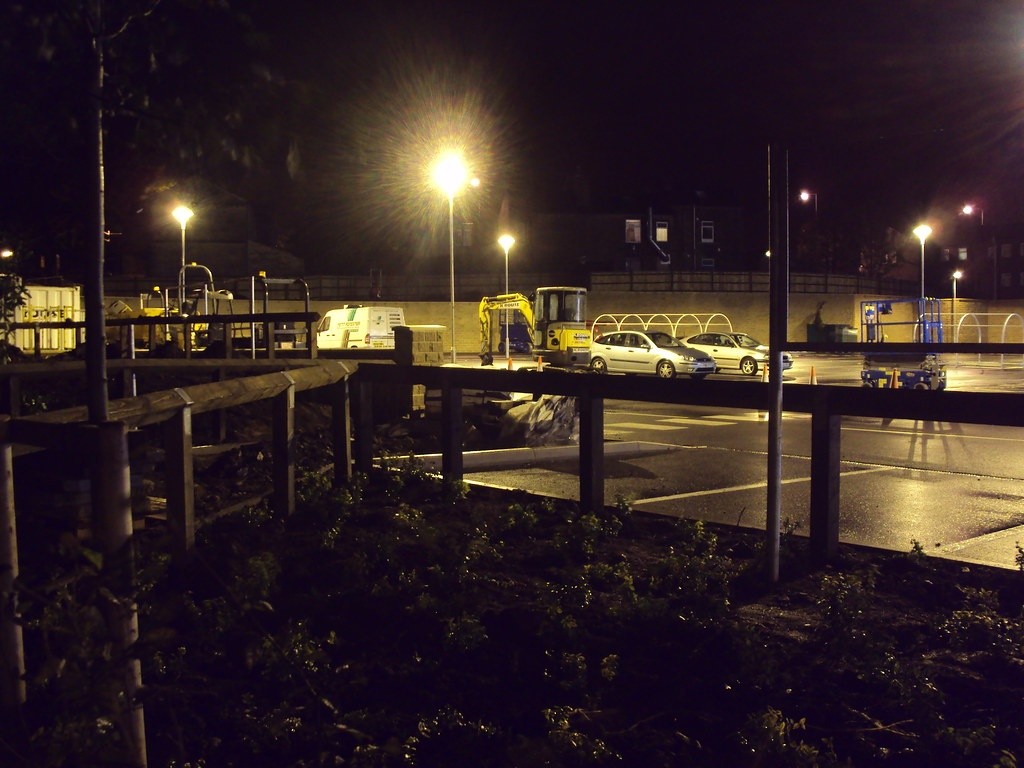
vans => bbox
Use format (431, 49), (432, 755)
(316, 304), (406, 350)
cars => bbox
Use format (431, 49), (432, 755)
(590, 330), (717, 380)
(672, 331), (795, 376)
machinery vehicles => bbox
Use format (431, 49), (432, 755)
(478, 285), (591, 371)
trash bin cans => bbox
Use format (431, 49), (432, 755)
(806, 323), (859, 343)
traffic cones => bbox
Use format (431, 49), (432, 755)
(507, 358), (514, 371)
(537, 356), (543, 373)
(761, 363), (770, 383)
(808, 365), (818, 386)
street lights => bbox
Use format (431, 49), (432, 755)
(913, 224), (932, 299)
(499, 233), (515, 369)
(951, 269), (964, 341)
(801, 191), (818, 216)
(171, 205), (194, 302)
(963, 205), (984, 225)
(432, 152), (465, 364)
